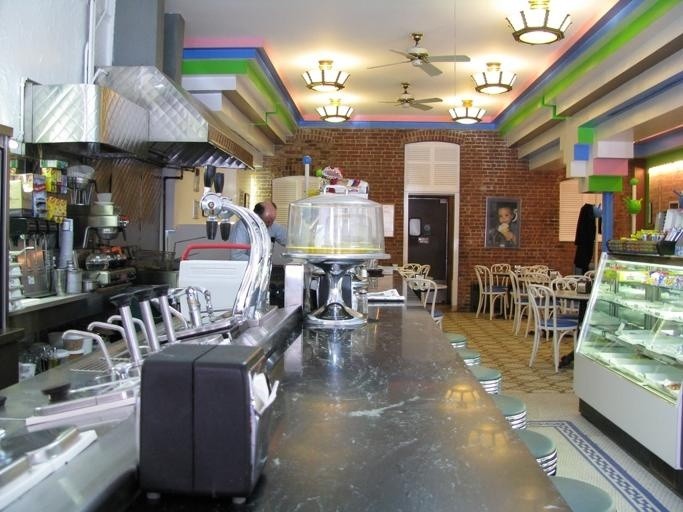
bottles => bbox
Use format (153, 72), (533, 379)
(357, 287), (368, 316)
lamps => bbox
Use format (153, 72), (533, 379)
(302, 69), (350, 92)
(470, 71), (516, 94)
(316, 105), (352, 123)
(505, 9), (572, 46)
(449, 108), (485, 124)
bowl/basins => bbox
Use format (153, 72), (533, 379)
(67, 165), (95, 177)
(97, 193), (112, 202)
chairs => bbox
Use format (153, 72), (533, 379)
(404, 264), (420, 272)
(380, 98), (443, 110)
(528, 272), (551, 288)
(406, 279), (443, 333)
(527, 265), (548, 284)
(528, 284), (578, 372)
(508, 270), (529, 336)
(492, 264), (510, 288)
(416, 265), (430, 278)
(564, 275), (592, 317)
(551, 278), (576, 316)
(474, 265), (508, 320)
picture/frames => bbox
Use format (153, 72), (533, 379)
(484, 197), (521, 250)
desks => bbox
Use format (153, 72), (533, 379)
(555, 289), (592, 368)
(409, 284), (447, 304)
(494, 271), (529, 320)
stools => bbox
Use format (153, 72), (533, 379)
(546, 476), (617, 512)
(446, 333), (466, 349)
(469, 366), (502, 394)
(487, 393), (527, 430)
(456, 348), (479, 366)
(513, 429), (556, 477)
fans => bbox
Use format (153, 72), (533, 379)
(366, 49), (470, 77)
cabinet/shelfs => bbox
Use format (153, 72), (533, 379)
(575, 253), (682, 488)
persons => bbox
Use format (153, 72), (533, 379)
(229, 201), (288, 261)
(491, 201), (519, 246)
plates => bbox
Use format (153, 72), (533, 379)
(93, 202), (115, 205)
